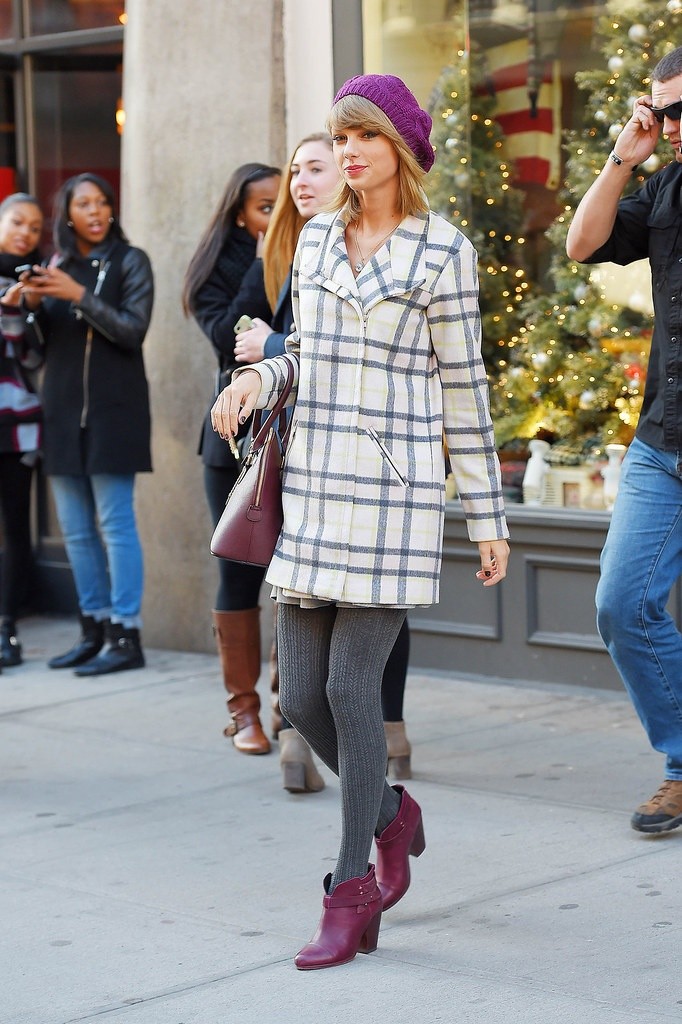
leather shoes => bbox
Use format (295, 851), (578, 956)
(0, 624), (23, 665)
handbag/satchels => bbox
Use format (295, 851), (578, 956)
(211, 355), (297, 567)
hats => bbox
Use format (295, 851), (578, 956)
(332, 74), (435, 173)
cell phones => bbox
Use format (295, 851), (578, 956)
(15, 263), (41, 279)
(233, 315), (256, 335)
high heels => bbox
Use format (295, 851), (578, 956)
(381, 719), (412, 780)
(295, 863), (382, 971)
(278, 729), (325, 793)
(376, 784), (427, 912)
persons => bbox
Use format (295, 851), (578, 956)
(182, 163), (283, 754)
(0, 192), (44, 673)
(566, 50), (682, 833)
(210, 74), (511, 969)
(19, 174), (153, 676)
(233, 133), (412, 792)
(470, 6), (554, 183)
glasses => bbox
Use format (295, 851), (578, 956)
(646, 100), (682, 124)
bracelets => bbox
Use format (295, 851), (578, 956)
(609, 152), (638, 171)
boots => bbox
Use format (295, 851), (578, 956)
(47, 613), (111, 668)
(268, 603), (294, 739)
(74, 623), (145, 676)
(211, 606), (271, 754)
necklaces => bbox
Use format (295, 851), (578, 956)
(354, 220), (400, 272)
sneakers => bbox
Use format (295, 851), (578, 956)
(631, 779), (682, 834)
